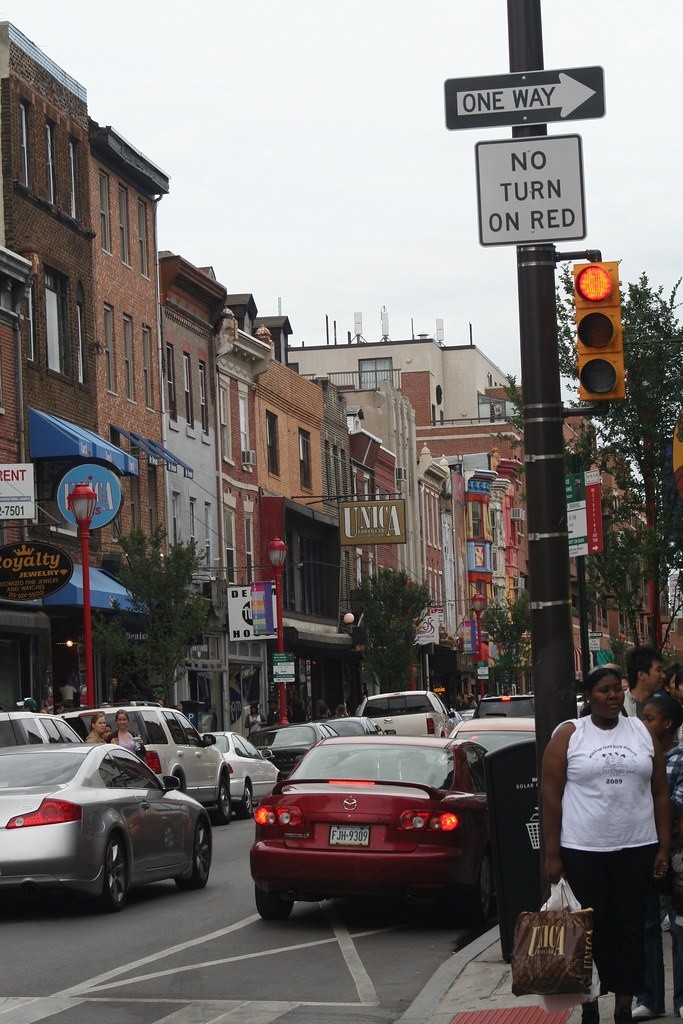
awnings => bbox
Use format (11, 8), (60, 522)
(29, 406), (138, 477)
(44, 563), (150, 615)
(110, 424), (193, 479)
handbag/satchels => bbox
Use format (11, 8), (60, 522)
(511, 875), (600, 1012)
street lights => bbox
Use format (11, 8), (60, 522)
(471, 593), (485, 698)
(265, 536), (290, 725)
(68, 482), (99, 709)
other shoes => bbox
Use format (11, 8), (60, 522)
(631, 1005), (655, 1018)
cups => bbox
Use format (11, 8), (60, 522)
(132, 737), (142, 750)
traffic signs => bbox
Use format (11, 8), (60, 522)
(588, 632), (603, 638)
(446, 65), (605, 130)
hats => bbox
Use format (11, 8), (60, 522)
(17, 698), (38, 710)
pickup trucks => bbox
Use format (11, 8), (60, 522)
(355, 690), (457, 765)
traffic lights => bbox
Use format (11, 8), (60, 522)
(573, 261), (626, 402)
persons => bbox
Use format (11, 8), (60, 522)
(155, 697), (164, 707)
(85, 710), (147, 763)
(456, 693), (477, 711)
(16, 682), (77, 714)
(542, 668), (671, 1024)
(622, 644), (683, 1024)
(244, 691), (368, 735)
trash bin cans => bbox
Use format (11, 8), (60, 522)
(482, 738), (538, 964)
(180, 700), (212, 733)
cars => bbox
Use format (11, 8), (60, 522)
(200, 731), (283, 820)
(321, 715), (405, 781)
(436, 718), (536, 766)
(249, 736), (496, 928)
(247, 722), (344, 780)
(0, 711), (84, 748)
(0, 743), (214, 915)
(450, 708), (475, 740)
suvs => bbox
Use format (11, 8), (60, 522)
(468, 691), (536, 741)
(54, 700), (232, 824)
